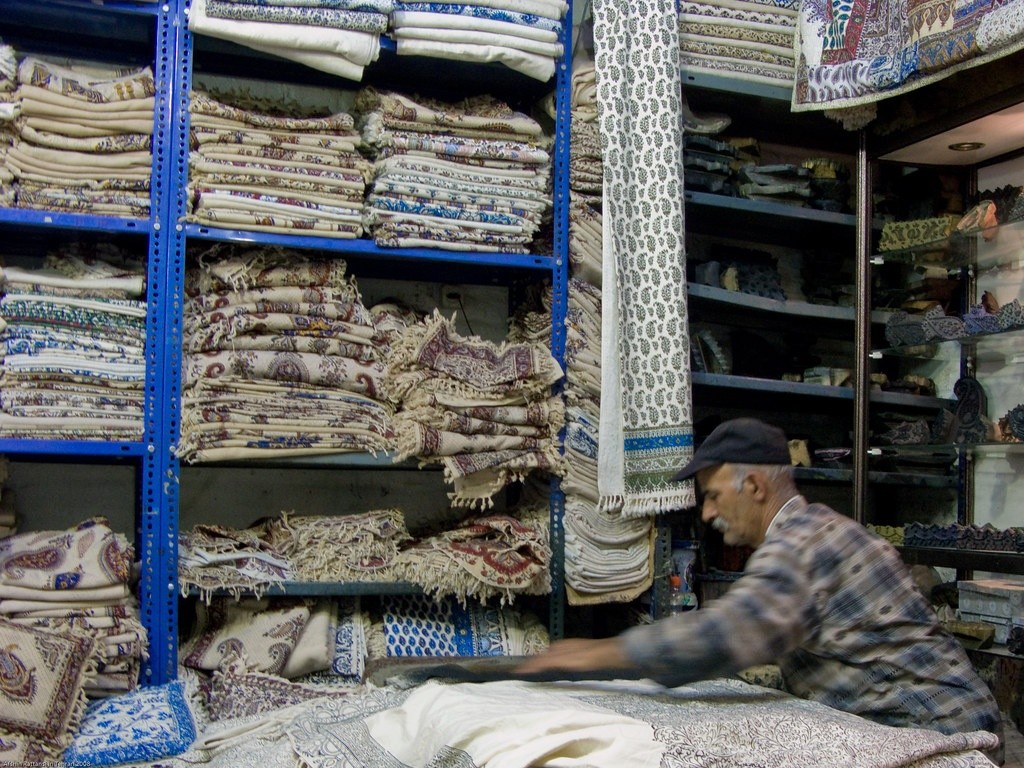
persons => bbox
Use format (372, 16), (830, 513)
(506, 419), (1007, 765)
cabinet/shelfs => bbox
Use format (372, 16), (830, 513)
(162, 0), (575, 686)
(853, 48), (1024, 655)
(649, 70), (979, 621)
(0, 0), (177, 688)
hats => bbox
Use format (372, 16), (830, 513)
(667, 418), (793, 482)
